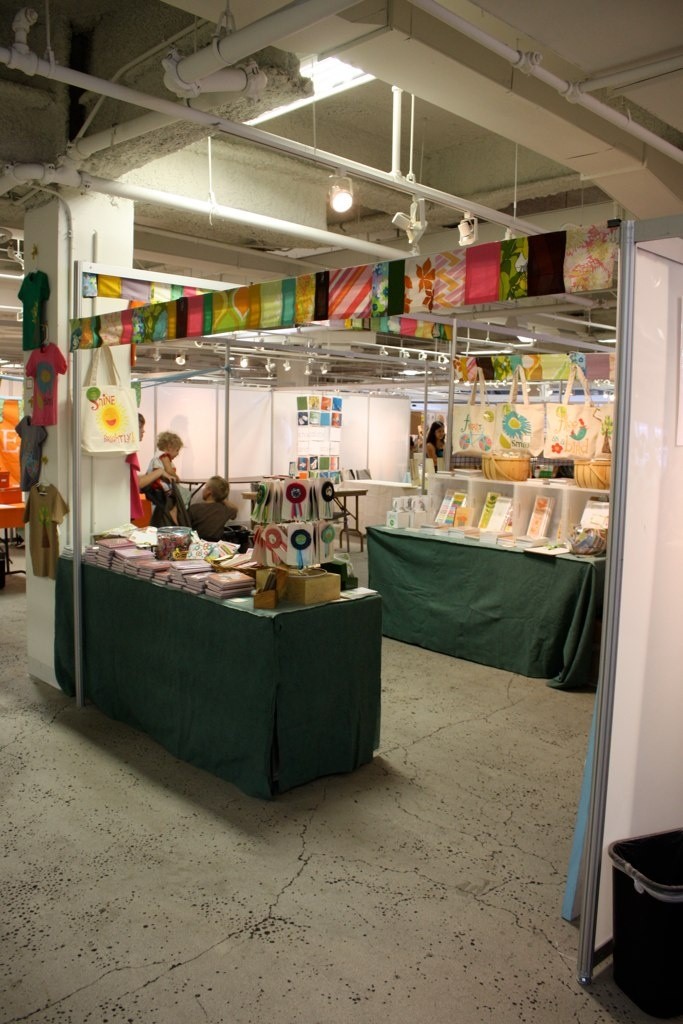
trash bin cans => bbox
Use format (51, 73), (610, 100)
(609, 829), (683, 1019)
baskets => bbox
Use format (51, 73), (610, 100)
(574, 460), (612, 490)
(481, 454), (530, 482)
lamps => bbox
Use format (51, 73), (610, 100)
(392, 195), (427, 246)
(458, 211), (479, 246)
(327, 167), (354, 214)
(150, 336), (449, 376)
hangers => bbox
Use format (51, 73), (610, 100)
(30, 252), (39, 273)
(36, 461), (49, 495)
(41, 324), (50, 345)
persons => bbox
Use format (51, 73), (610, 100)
(186, 476), (238, 542)
(136, 414), (184, 526)
(425, 421), (445, 472)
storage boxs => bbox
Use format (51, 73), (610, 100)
(286, 571), (341, 606)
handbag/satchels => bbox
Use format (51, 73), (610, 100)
(79, 346), (139, 457)
(494, 365), (544, 457)
(543, 364), (605, 460)
(451, 367), (496, 457)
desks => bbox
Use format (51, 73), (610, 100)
(54, 556), (382, 801)
(345, 480), (422, 536)
(366, 524), (607, 689)
(241, 489), (368, 552)
(178, 475), (270, 507)
(0, 501), (26, 576)
